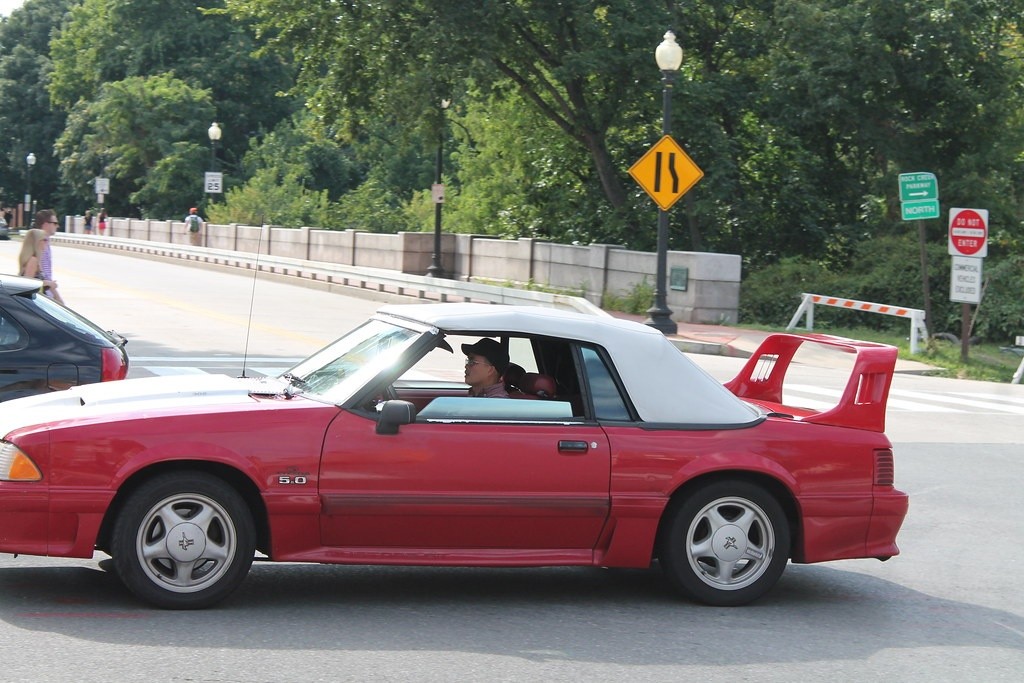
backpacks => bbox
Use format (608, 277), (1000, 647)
(189, 216), (199, 232)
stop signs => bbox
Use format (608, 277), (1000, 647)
(950, 210), (987, 255)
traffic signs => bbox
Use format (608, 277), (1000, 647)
(898, 172), (938, 201)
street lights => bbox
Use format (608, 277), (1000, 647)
(204, 120), (224, 221)
(425, 93), (449, 278)
(20, 152), (37, 228)
(643, 25), (687, 336)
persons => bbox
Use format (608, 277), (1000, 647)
(97, 208), (108, 236)
(184, 208), (203, 246)
(448, 338), (512, 416)
(83, 210), (92, 234)
(19, 209), (63, 304)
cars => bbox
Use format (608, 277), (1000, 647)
(0, 256), (130, 401)
(0, 305), (908, 613)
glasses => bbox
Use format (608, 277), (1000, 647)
(47, 221), (59, 225)
(464, 358), (491, 367)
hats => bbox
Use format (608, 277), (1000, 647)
(461, 338), (510, 375)
(189, 208), (197, 211)
